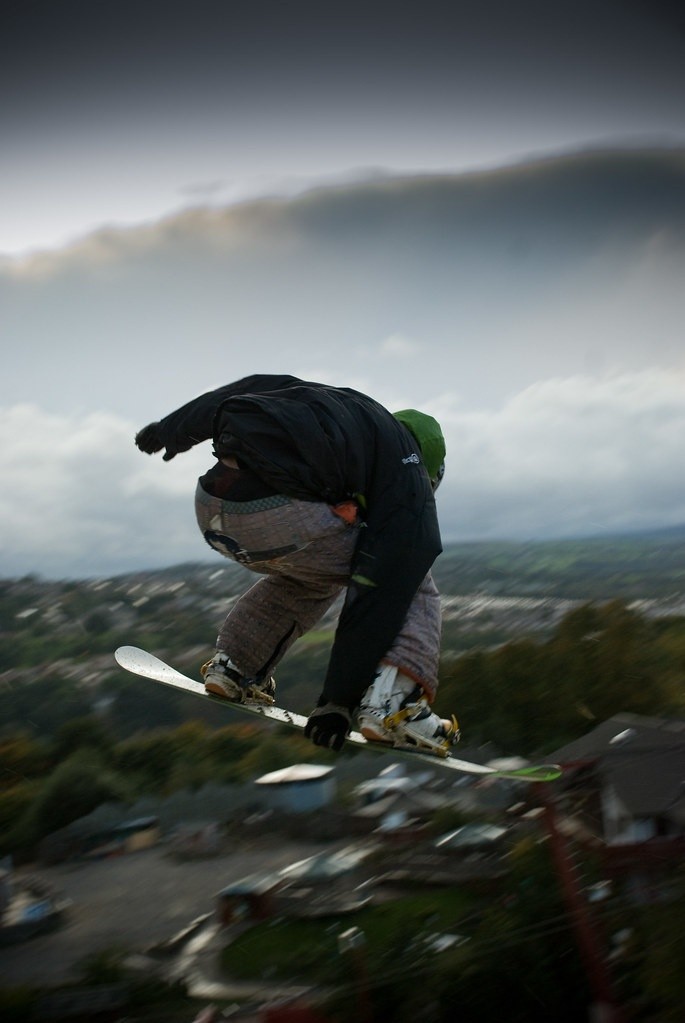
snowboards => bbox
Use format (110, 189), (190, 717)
(115, 644), (564, 786)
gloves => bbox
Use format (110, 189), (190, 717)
(303, 702), (354, 753)
(135, 421), (197, 460)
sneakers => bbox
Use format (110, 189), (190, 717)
(358, 661), (461, 758)
(200, 646), (275, 706)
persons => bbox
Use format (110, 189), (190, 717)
(132, 371), (461, 757)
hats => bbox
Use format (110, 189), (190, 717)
(393, 409), (445, 487)
